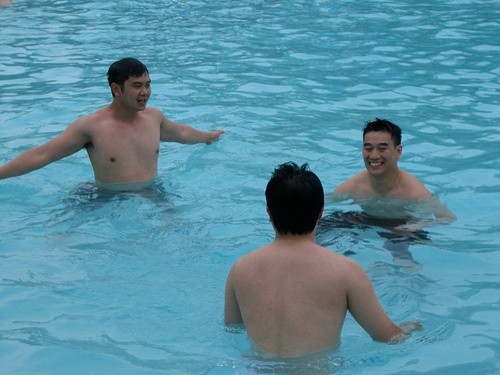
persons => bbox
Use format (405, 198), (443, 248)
(0, 58), (225, 190)
(223, 160), (424, 360)
(324, 118), (456, 269)
